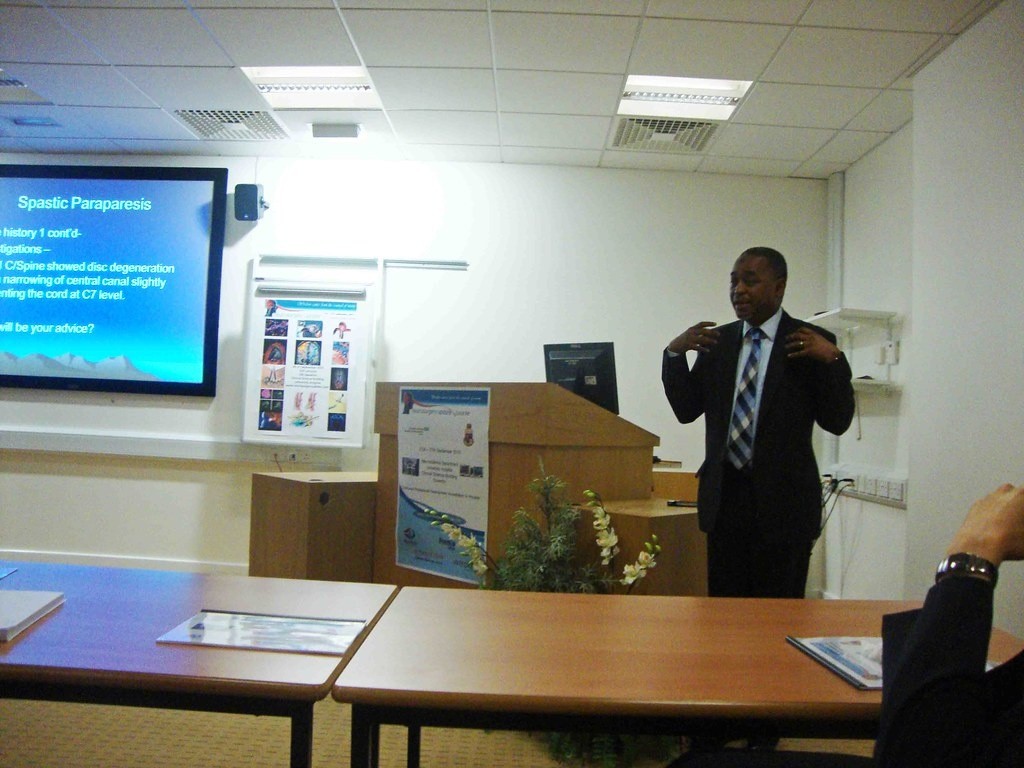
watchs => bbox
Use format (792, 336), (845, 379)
(830, 349), (841, 362)
(936, 552), (1001, 585)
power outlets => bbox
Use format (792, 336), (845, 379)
(824, 471), (838, 486)
(846, 474), (859, 492)
(877, 479), (889, 498)
(865, 477), (878, 495)
(889, 482), (904, 502)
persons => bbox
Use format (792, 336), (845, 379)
(872, 482), (1024, 768)
(662, 248), (855, 750)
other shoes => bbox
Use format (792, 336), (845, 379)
(749, 737), (781, 753)
(691, 735), (725, 750)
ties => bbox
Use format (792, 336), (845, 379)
(726, 327), (763, 470)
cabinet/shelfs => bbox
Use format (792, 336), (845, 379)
(805, 305), (902, 397)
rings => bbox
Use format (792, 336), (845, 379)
(698, 335), (703, 338)
(799, 340), (804, 346)
(697, 343), (700, 347)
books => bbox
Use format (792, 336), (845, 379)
(0, 590), (67, 643)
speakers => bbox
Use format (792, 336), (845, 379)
(234, 184), (265, 221)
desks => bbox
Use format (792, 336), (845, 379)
(332, 583), (1024, 768)
(0, 560), (399, 768)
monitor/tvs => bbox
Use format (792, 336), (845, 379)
(544, 341), (620, 416)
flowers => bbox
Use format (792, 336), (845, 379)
(424, 455), (662, 595)
